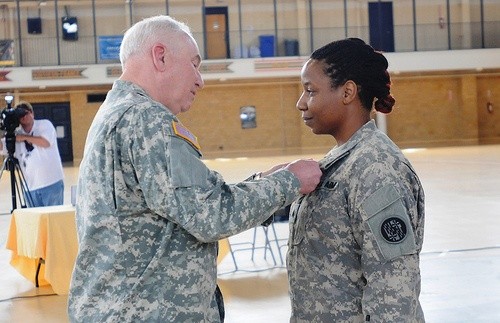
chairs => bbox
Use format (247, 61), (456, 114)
(217, 205), (292, 276)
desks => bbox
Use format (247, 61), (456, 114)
(5, 205), (238, 295)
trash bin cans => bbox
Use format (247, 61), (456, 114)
(259, 32), (275, 58)
(284, 39), (299, 57)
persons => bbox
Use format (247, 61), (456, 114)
(67, 15), (322, 323)
(0, 102), (64, 207)
(285, 37), (426, 323)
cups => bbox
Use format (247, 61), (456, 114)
(71, 185), (77, 206)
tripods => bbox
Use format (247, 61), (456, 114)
(0, 156), (36, 214)
